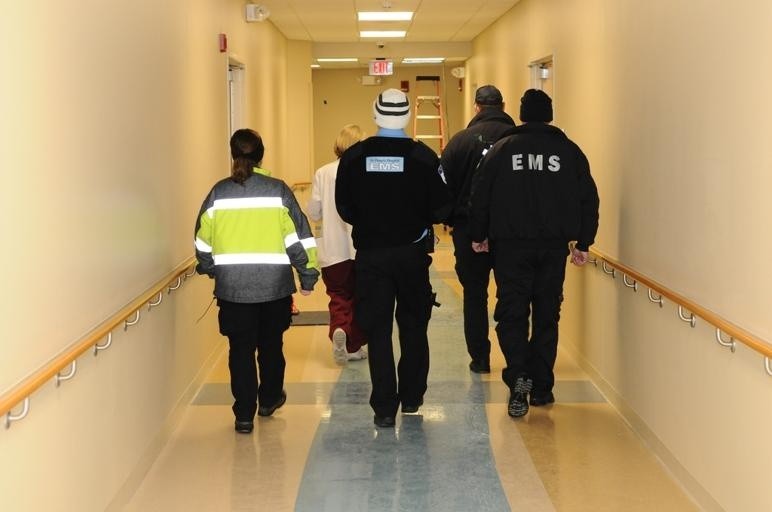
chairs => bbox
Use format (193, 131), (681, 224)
(373, 415), (396, 427)
(469, 359), (491, 374)
(346, 338), (368, 362)
(401, 396), (423, 413)
(257, 389), (287, 417)
(234, 415), (255, 434)
(508, 372), (532, 417)
(529, 390), (555, 407)
(333, 328), (349, 366)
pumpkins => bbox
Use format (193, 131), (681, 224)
(413, 76), (448, 233)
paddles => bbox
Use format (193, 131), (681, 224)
(289, 310), (332, 327)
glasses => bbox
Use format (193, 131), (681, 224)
(247, 3), (271, 22)
(374, 75), (382, 85)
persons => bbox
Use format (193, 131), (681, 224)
(442, 84), (518, 373)
(334, 87), (456, 428)
(305, 123), (370, 364)
(193, 130), (319, 434)
(469, 90), (600, 418)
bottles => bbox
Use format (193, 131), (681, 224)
(519, 88), (553, 122)
(372, 88), (411, 129)
(475, 85), (503, 104)
